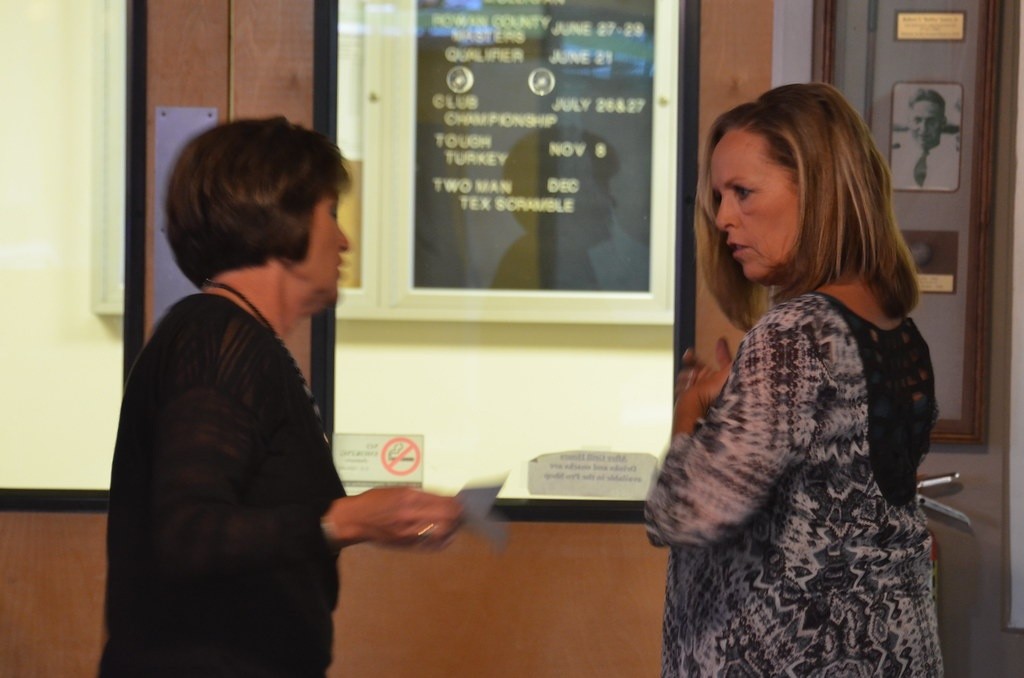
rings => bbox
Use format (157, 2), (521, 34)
(418, 521), (439, 540)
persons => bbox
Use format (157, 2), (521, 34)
(97, 116), (464, 678)
(893, 90), (959, 190)
(642, 82), (942, 678)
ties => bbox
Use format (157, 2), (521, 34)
(912, 147), (928, 187)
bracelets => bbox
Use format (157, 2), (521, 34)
(322, 514), (336, 543)
